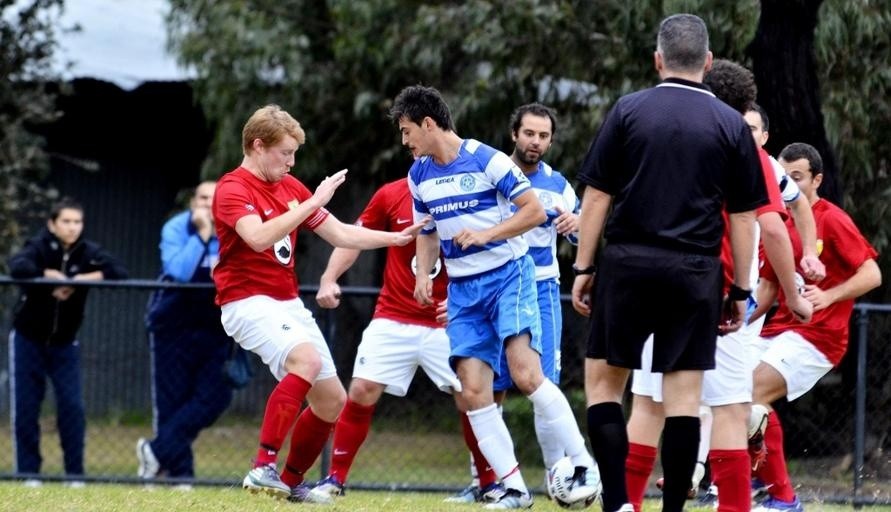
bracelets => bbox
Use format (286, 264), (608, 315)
(729, 284), (751, 300)
(572, 266), (594, 275)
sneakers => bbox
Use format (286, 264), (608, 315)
(689, 485), (719, 510)
(750, 475), (770, 497)
(135, 436), (161, 493)
(241, 462), (345, 505)
(756, 493), (803, 511)
(482, 481), (508, 501)
(655, 476), (699, 499)
(485, 490), (535, 511)
(443, 484), (481, 504)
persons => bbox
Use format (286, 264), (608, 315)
(9, 199), (130, 488)
(135, 179), (233, 491)
(747, 145), (882, 511)
(625, 61), (814, 512)
(312, 179), (505, 505)
(656, 102), (826, 498)
(443, 102), (583, 503)
(388, 85), (592, 510)
(212, 105), (432, 504)
(570, 15), (770, 512)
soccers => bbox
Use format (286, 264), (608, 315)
(547, 456), (600, 511)
